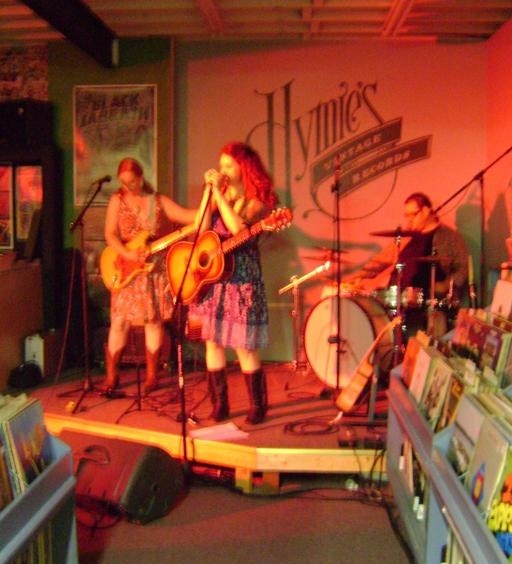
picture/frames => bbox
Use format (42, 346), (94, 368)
(73, 84), (158, 207)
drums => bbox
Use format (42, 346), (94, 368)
(390, 283), (424, 309)
(302, 294), (397, 389)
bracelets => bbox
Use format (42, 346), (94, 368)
(206, 181), (213, 185)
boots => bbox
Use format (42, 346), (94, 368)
(242, 369), (267, 424)
(144, 346), (160, 391)
(101, 346), (124, 390)
(206, 369), (229, 421)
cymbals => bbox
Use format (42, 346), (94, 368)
(410, 256), (449, 261)
(369, 231), (423, 236)
(302, 244), (350, 265)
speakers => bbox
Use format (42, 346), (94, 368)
(57, 427), (188, 523)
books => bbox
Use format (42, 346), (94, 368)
(0, 385), (63, 562)
(395, 256), (512, 563)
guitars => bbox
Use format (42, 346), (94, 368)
(101, 225), (199, 293)
(334, 316), (403, 413)
(166, 207), (294, 306)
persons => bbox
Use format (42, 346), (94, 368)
(97, 157), (202, 397)
(194, 140), (283, 424)
(388, 192), (466, 330)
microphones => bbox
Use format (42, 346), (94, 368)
(93, 174), (112, 184)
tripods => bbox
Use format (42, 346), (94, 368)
(54, 193), (105, 415)
(267, 288), (311, 389)
(108, 313), (167, 424)
